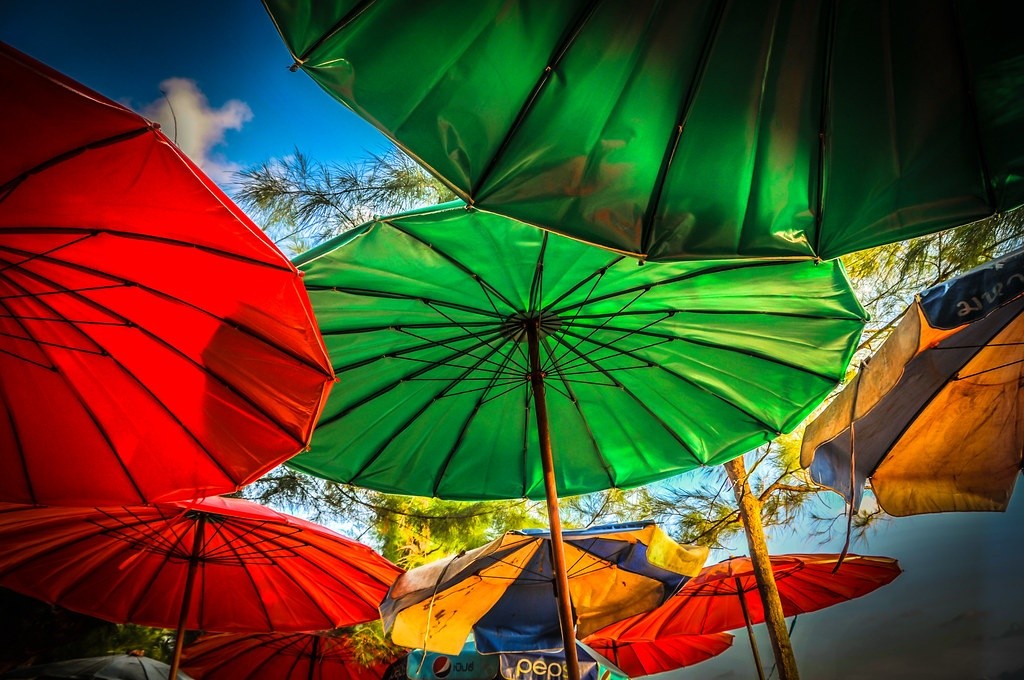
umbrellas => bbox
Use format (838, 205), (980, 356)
(0, 0), (1024, 680)
